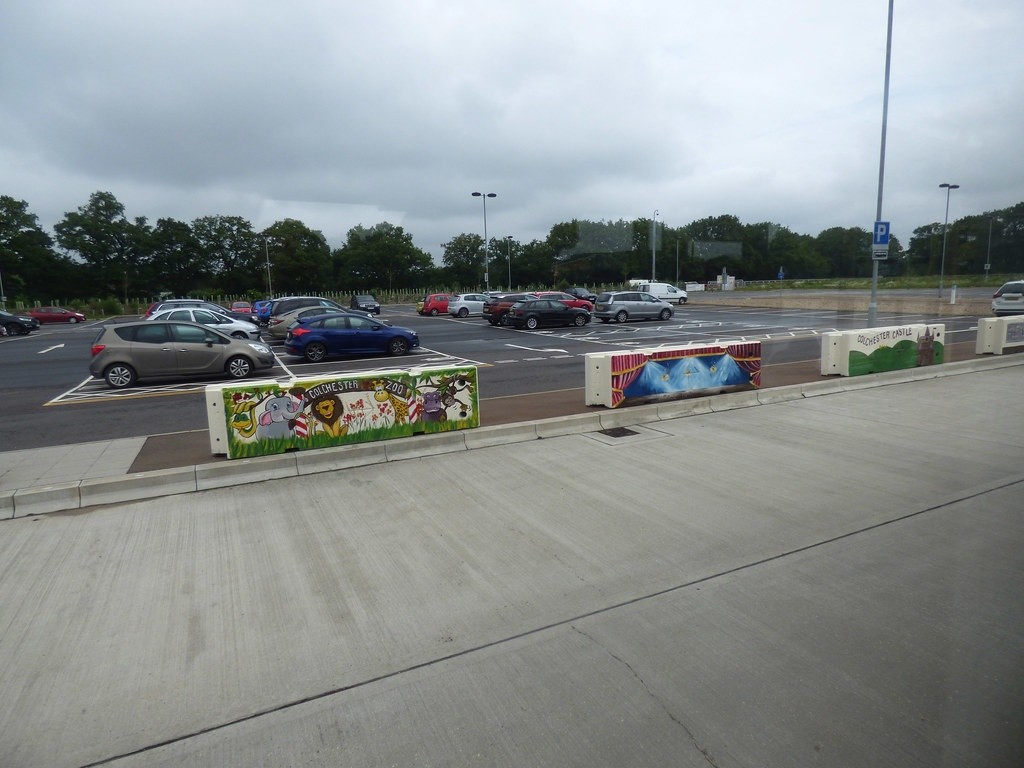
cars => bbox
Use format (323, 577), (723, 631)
(0, 310), (41, 335)
(144, 292), (374, 343)
(507, 299), (592, 329)
(415, 286), (600, 323)
(0, 325), (8, 336)
(992, 281), (1024, 315)
(268, 307), (381, 338)
(350, 294), (381, 314)
(27, 306), (87, 324)
(284, 313), (421, 364)
(88, 322), (276, 388)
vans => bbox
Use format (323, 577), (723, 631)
(594, 291), (675, 322)
(637, 284), (688, 304)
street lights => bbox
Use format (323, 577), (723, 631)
(472, 192), (497, 296)
(938, 184), (960, 299)
(652, 209), (659, 283)
